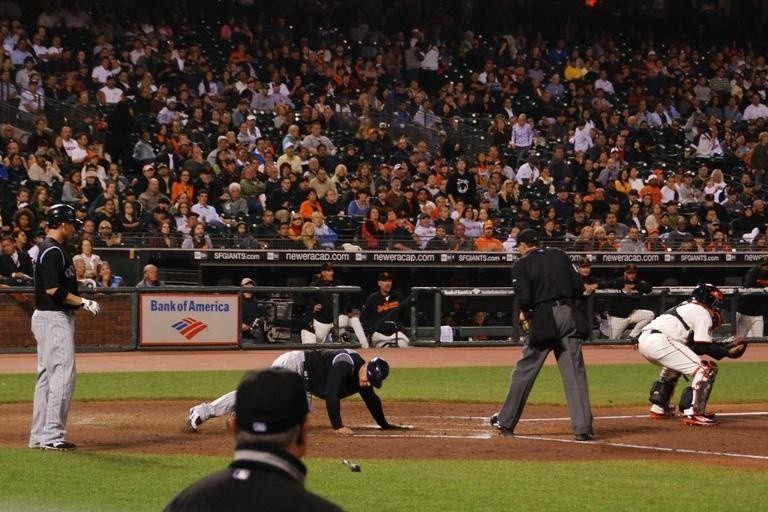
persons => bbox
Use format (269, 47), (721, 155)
(491, 228), (595, 441)
(638, 285), (744, 425)
(162, 369), (345, 512)
(188, 346), (415, 436)
(0, 0), (767, 348)
(28, 205), (100, 450)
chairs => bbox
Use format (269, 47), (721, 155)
(0, 1), (767, 348)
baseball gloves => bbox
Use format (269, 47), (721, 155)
(726, 337), (748, 358)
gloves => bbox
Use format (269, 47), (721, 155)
(76, 296), (103, 318)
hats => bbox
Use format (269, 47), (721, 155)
(366, 357), (391, 389)
(44, 202), (84, 228)
(758, 255), (768, 267)
(320, 261), (335, 272)
(377, 272), (393, 282)
(625, 264), (637, 272)
(511, 228), (541, 249)
(692, 282), (725, 313)
(578, 258), (591, 268)
(240, 276), (256, 286)
(28, 78), (768, 239)
(233, 367), (311, 438)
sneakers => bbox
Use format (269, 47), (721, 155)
(186, 404), (203, 434)
(487, 412), (516, 437)
(649, 405), (678, 421)
(40, 439), (78, 454)
(574, 428), (595, 442)
(683, 414), (719, 427)
